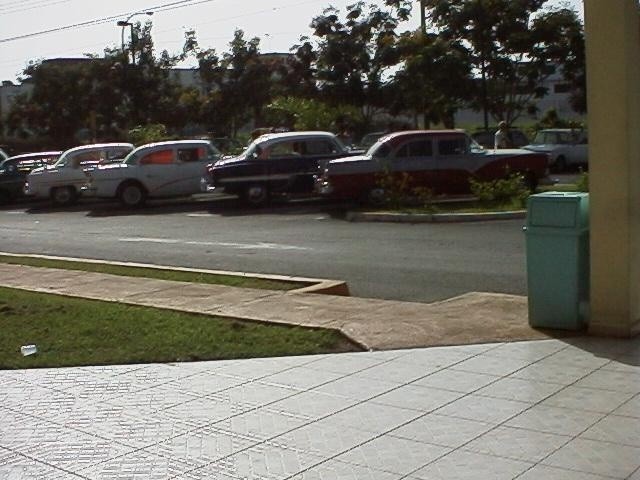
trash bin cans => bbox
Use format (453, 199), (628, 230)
(521, 191), (590, 332)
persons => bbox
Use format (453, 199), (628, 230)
(494, 120), (517, 149)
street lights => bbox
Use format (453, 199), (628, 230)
(123, 12), (154, 58)
(117, 22), (138, 122)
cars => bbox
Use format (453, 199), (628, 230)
(348, 131), (387, 151)
(520, 127), (589, 172)
(22, 141), (136, 207)
(207, 130), (367, 207)
(0, 150), (63, 193)
(314, 131), (548, 204)
(77, 140), (236, 207)
